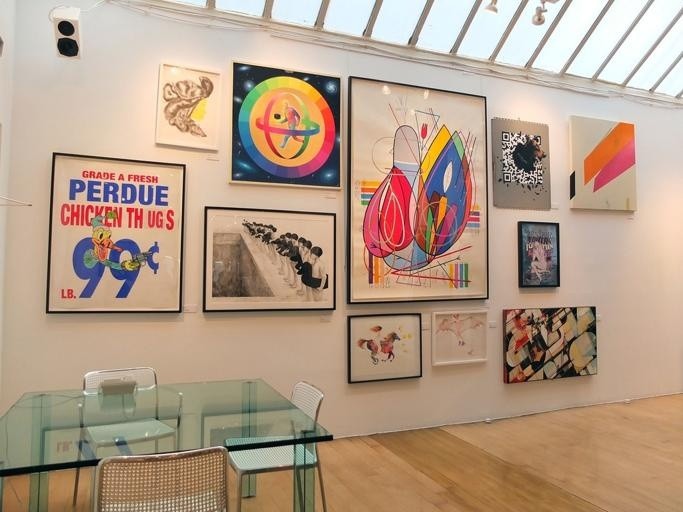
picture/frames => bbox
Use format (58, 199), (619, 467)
(155, 58), (224, 154)
(431, 311), (491, 368)
(228, 60), (345, 193)
(198, 406), (300, 450)
(38, 415), (181, 467)
(514, 220), (562, 290)
(344, 311), (424, 384)
(45, 149), (187, 316)
(344, 75), (491, 305)
(199, 205), (338, 315)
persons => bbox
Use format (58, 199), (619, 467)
(275, 100), (304, 149)
(527, 236), (553, 283)
(435, 316), (483, 347)
(241, 219), (327, 302)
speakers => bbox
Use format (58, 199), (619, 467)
(52, 5), (82, 62)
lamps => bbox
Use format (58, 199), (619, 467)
(482, 0), (498, 15)
(531, 0), (559, 25)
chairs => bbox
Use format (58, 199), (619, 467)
(69, 365), (182, 512)
(89, 442), (232, 512)
(224, 377), (329, 512)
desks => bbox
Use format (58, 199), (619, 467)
(0, 390), (330, 512)
